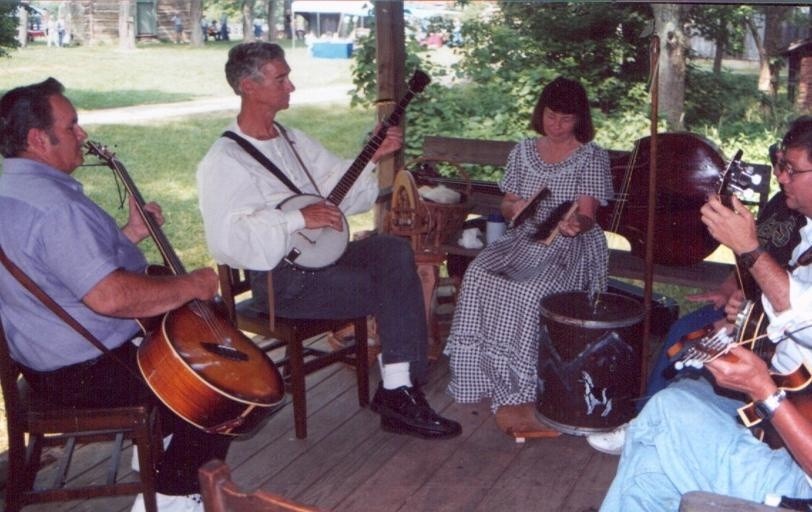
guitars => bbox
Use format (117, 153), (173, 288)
(674, 299), (767, 388)
(84, 138), (285, 440)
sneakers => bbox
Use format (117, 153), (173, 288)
(586, 421), (627, 455)
(131, 489), (205, 512)
(369, 377), (430, 414)
(130, 434), (171, 474)
(380, 386), (463, 442)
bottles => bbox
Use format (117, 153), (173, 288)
(484, 215), (507, 246)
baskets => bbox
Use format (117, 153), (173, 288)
(400, 158), (476, 245)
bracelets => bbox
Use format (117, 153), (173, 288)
(736, 244), (765, 273)
(42, 9), (308, 47)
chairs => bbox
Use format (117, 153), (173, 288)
(0, 309), (175, 511)
(195, 458), (334, 512)
(215, 263), (372, 442)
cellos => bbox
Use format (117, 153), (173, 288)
(406, 130), (731, 263)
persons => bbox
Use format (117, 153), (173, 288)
(0, 73), (237, 511)
(442, 78), (619, 413)
(594, 112), (812, 510)
(583, 140), (809, 459)
(195, 43), (463, 443)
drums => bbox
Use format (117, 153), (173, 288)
(535, 291), (645, 437)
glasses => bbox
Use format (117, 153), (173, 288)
(778, 159), (811, 177)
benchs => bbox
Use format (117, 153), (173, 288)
(413, 133), (774, 332)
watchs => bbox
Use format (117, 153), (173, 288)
(751, 385), (786, 426)
